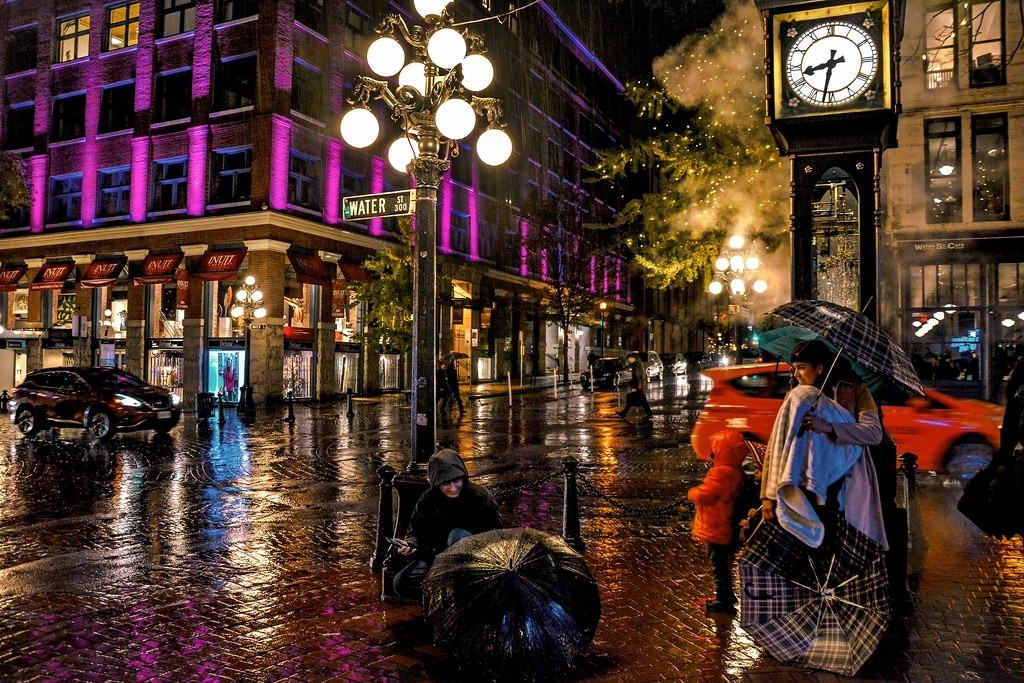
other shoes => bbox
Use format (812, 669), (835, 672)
(616, 411), (625, 417)
(643, 414), (653, 419)
(707, 597), (737, 611)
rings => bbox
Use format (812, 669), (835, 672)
(807, 420), (811, 425)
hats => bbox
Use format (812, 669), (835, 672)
(791, 339), (832, 364)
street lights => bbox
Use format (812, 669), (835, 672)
(708, 235), (768, 365)
(340, 1), (512, 604)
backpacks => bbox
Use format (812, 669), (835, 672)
(722, 465), (760, 547)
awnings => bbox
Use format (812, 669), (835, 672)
(286, 249), (329, 287)
(0, 264), (29, 292)
(80, 256), (130, 289)
(338, 260), (372, 283)
(31, 260), (77, 291)
(134, 251), (185, 286)
(192, 247), (248, 283)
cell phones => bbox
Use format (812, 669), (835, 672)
(384, 537), (412, 550)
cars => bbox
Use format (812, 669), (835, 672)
(7, 366), (183, 441)
(580, 356), (633, 390)
(712, 347), (763, 366)
(625, 350), (664, 384)
(690, 360), (1005, 487)
(683, 350), (711, 373)
(659, 352), (688, 378)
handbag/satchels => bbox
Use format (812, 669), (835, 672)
(626, 387), (643, 405)
(957, 455), (1024, 539)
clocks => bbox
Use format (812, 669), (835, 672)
(784, 21), (878, 109)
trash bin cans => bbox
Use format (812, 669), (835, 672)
(195, 392), (214, 418)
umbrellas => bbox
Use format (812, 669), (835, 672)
(738, 503), (895, 677)
(750, 294), (926, 438)
(440, 351), (470, 363)
(956, 464), (1024, 540)
(422, 527), (602, 683)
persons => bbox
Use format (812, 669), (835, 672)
(223, 357), (236, 401)
(436, 360), (468, 416)
(586, 349), (596, 368)
(759, 340), (914, 617)
(616, 352), (654, 419)
(908, 346), (1024, 475)
(688, 428), (763, 609)
(392, 448), (503, 601)
